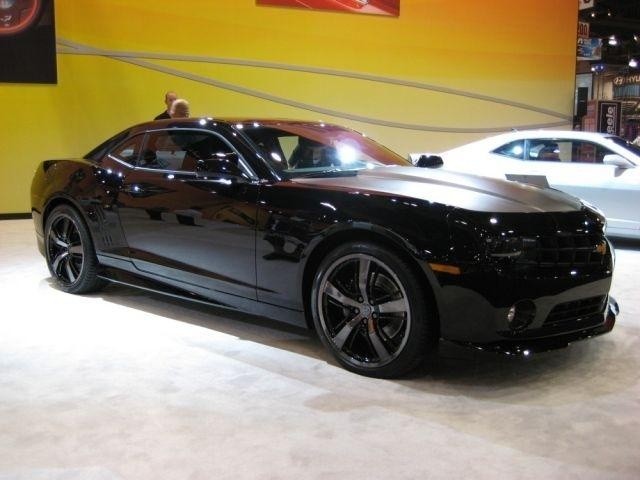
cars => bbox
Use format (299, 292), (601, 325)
(408, 127), (640, 241)
(30, 117), (619, 380)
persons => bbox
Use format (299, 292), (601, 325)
(169, 99), (190, 119)
(154, 92), (176, 120)
